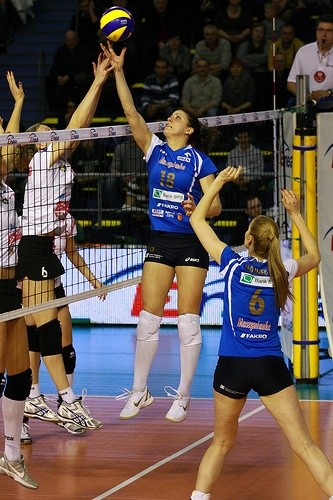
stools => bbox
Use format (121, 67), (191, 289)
(40, 82), (273, 230)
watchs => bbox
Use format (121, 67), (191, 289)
(328, 89), (333, 96)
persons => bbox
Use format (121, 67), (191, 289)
(16, 211), (107, 445)
(98, 41), (223, 423)
(0, 0), (333, 246)
(21, 52), (115, 432)
(0, 70), (40, 489)
(186, 165), (333, 500)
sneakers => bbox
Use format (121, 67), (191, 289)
(0, 453), (39, 489)
(20, 423), (32, 445)
(54, 422), (87, 435)
(164, 386), (190, 422)
(24, 394), (61, 422)
(115, 385), (154, 420)
(56, 388), (102, 431)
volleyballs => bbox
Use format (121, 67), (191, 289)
(100, 5), (135, 41)
